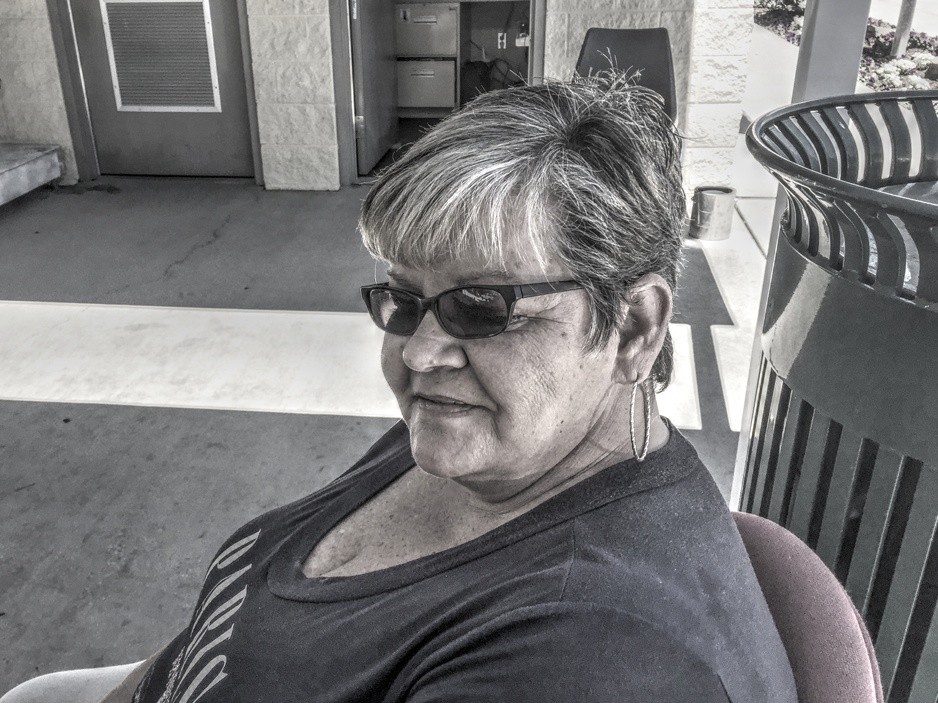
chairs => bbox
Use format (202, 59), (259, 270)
(572, 28), (678, 130)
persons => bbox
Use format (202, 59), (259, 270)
(0, 47), (799, 703)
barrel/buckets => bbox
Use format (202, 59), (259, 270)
(462, 61), (490, 101)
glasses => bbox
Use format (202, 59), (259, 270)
(360, 274), (578, 340)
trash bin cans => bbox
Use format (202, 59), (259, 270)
(745, 88), (937, 703)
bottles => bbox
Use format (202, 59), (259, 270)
(519, 24), (527, 38)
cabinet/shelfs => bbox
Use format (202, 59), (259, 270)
(395, 1), (472, 118)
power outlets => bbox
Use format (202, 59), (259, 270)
(498, 33), (506, 50)
